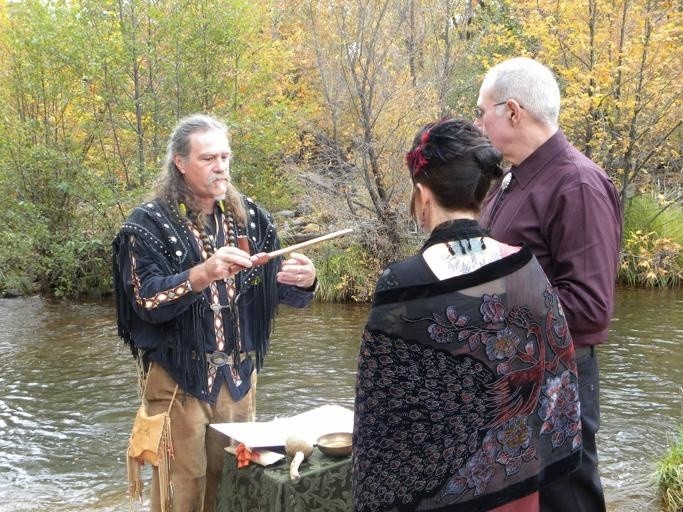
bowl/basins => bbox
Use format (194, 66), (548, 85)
(315, 432), (358, 459)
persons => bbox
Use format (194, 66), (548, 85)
(108, 114), (326, 512)
(349, 115), (586, 512)
(471, 55), (627, 512)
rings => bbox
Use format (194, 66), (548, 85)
(297, 273), (302, 282)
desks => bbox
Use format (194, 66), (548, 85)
(218, 446), (355, 512)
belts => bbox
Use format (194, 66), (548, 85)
(575, 346), (591, 359)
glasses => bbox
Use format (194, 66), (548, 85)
(474, 102), (524, 118)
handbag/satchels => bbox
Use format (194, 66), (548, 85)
(129, 401), (171, 466)
